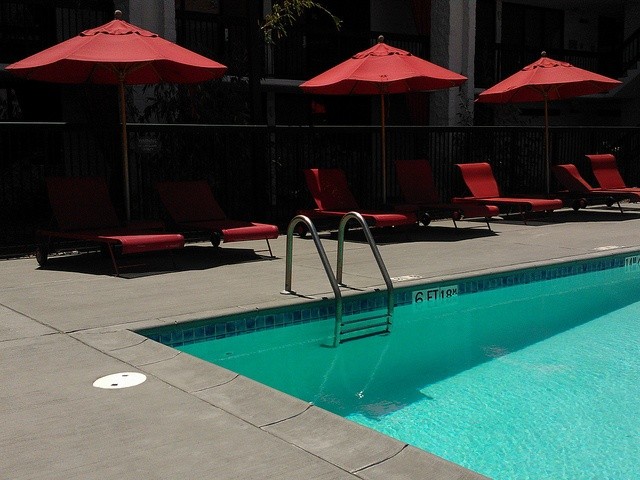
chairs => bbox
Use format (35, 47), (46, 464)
(392, 159), (499, 232)
(152, 179), (280, 257)
(548, 163), (640, 214)
(36, 172), (184, 277)
(296, 167), (419, 241)
(585, 153), (640, 208)
(448, 162), (564, 226)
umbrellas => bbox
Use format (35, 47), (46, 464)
(0, 9), (227, 222)
(475, 50), (624, 198)
(298, 35), (467, 207)
